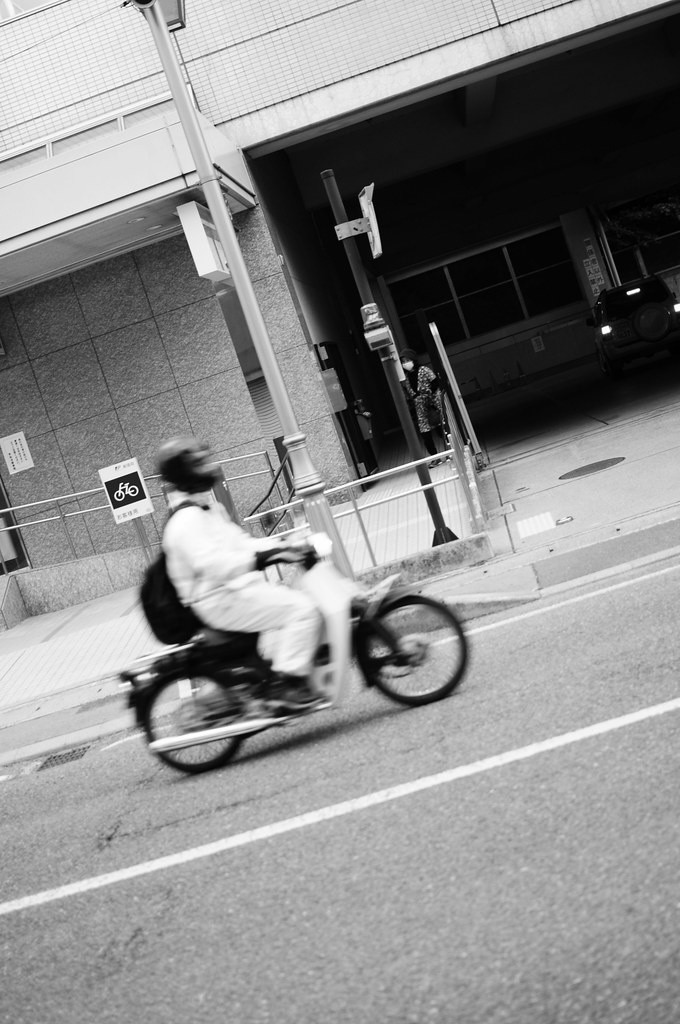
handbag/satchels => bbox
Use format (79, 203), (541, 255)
(424, 391), (443, 427)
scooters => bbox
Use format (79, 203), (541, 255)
(119, 531), (469, 774)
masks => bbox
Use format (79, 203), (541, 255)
(401, 361), (414, 371)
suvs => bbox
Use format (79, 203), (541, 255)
(586, 272), (680, 380)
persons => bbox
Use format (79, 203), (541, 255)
(158, 434), (333, 713)
(398, 348), (453, 469)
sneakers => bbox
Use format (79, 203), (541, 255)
(442, 456), (451, 463)
(427, 458), (443, 469)
(260, 683), (335, 714)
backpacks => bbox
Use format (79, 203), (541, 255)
(139, 500), (212, 646)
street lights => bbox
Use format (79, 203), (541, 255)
(119, 0), (357, 584)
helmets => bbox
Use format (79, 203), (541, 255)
(155, 435), (226, 494)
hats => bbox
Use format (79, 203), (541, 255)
(399, 348), (419, 363)
